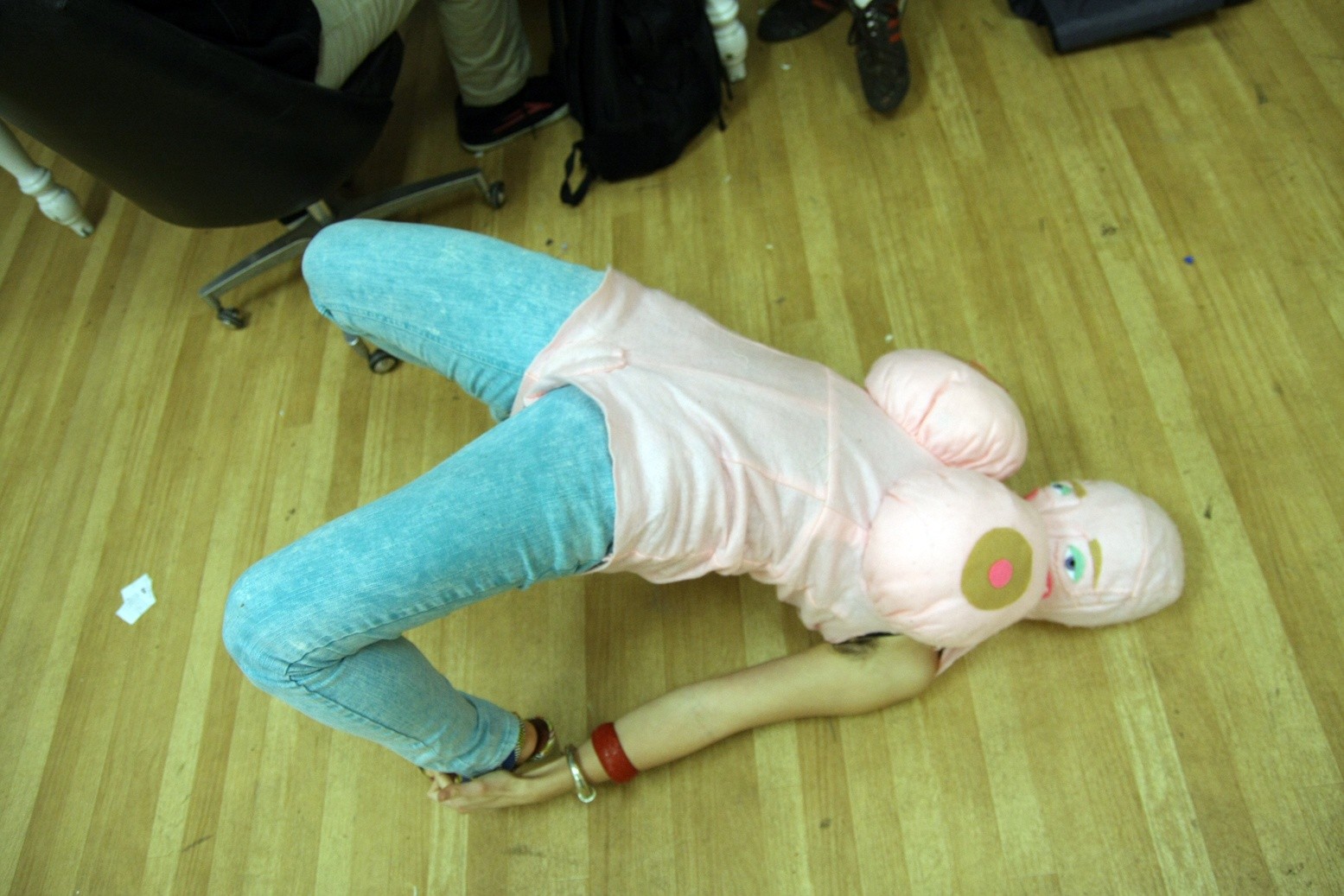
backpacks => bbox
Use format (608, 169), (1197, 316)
(559, 0), (734, 210)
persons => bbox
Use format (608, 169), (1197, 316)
(220, 217), (1190, 816)
(0, 0), (569, 201)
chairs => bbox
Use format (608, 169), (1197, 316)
(0, 0), (507, 375)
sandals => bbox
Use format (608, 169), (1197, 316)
(418, 715), (557, 785)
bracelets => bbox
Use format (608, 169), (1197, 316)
(590, 722), (641, 784)
(563, 744), (597, 803)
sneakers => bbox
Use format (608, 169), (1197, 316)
(454, 73), (569, 153)
(848, 0), (912, 117)
(757, 0), (848, 45)
